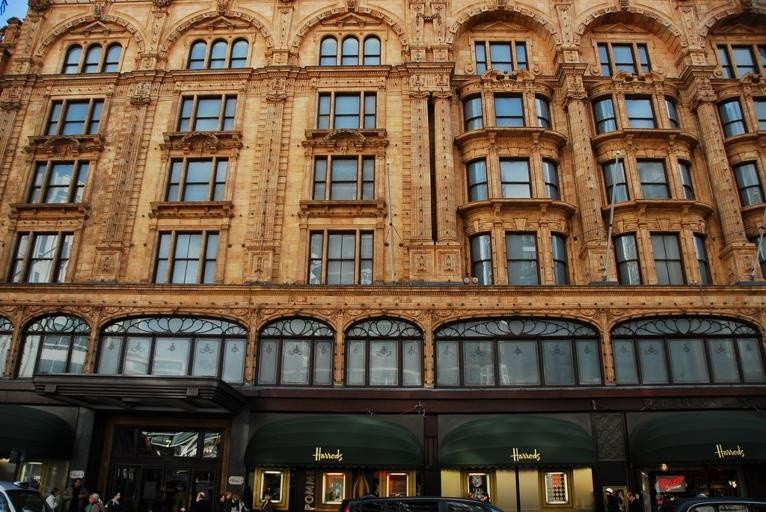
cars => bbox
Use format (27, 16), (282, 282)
(0, 481), (52, 511)
(657, 498), (766, 512)
(339, 497), (503, 512)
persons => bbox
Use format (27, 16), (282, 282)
(152, 486), (210, 512)
(392, 488), (401, 497)
(663, 493), (679, 512)
(272, 488), (279, 500)
(695, 490), (710, 498)
(606, 490), (642, 512)
(469, 476), (484, 495)
(260, 494), (273, 512)
(478, 493), (491, 504)
(31, 474), (121, 512)
(329, 491), (338, 501)
(214, 491), (249, 512)
